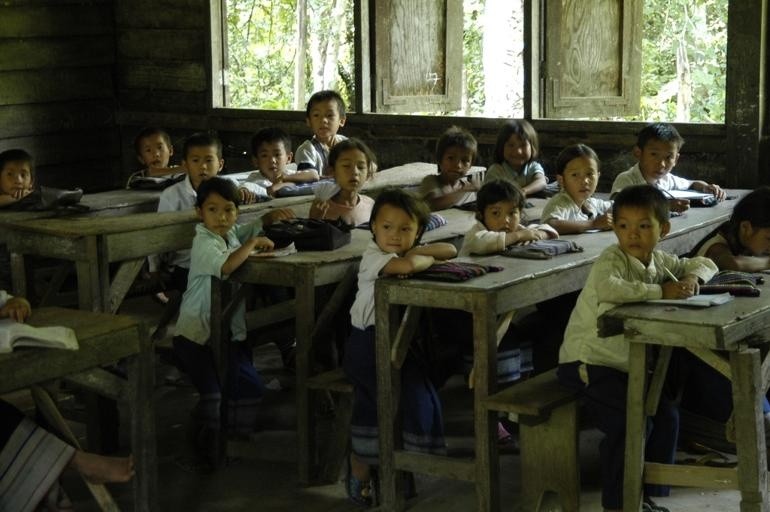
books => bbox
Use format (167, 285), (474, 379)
(0, 322), (80, 353)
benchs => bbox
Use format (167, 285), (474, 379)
(482, 369), (592, 511)
(242, 298), (298, 337)
(304, 360), (369, 485)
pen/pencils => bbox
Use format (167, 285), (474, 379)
(663, 266), (679, 282)
(655, 182), (676, 199)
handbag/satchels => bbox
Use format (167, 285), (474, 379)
(273, 177), (336, 198)
(0, 186), (83, 212)
(662, 197), (718, 208)
(356, 214), (447, 232)
(700, 270), (765, 297)
(407, 262), (503, 281)
(129, 173), (186, 192)
(498, 239), (584, 259)
(526, 180), (561, 199)
(450, 201), (535, 211)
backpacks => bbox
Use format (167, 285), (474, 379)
(262, 216), (355, 252)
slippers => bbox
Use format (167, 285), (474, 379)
(402, 441), (455, 458)
(604, 502), (670, 512)
(343, 450), (373, 506)
(469, 422), (515, 450)
(675, 452), (738, 467)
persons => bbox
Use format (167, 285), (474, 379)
(556, 184), (719, 511)
(0, 149), (41, 309)
(672, 187), (768, 454)
(341, 187), (458, 508)
(0, 289), (138, 512)
(421, 118), (725, 446)
(128, 91), (379, 403)
(172, 177), (296, 480)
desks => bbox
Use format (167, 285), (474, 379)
(210, 194), (615, 462)
(0, 163), (295, 222)
(7, 161), (488, 312)
(597, 265), (769, 511)
(0, 302), (163, 512)
(375, 182), (754, 508)
